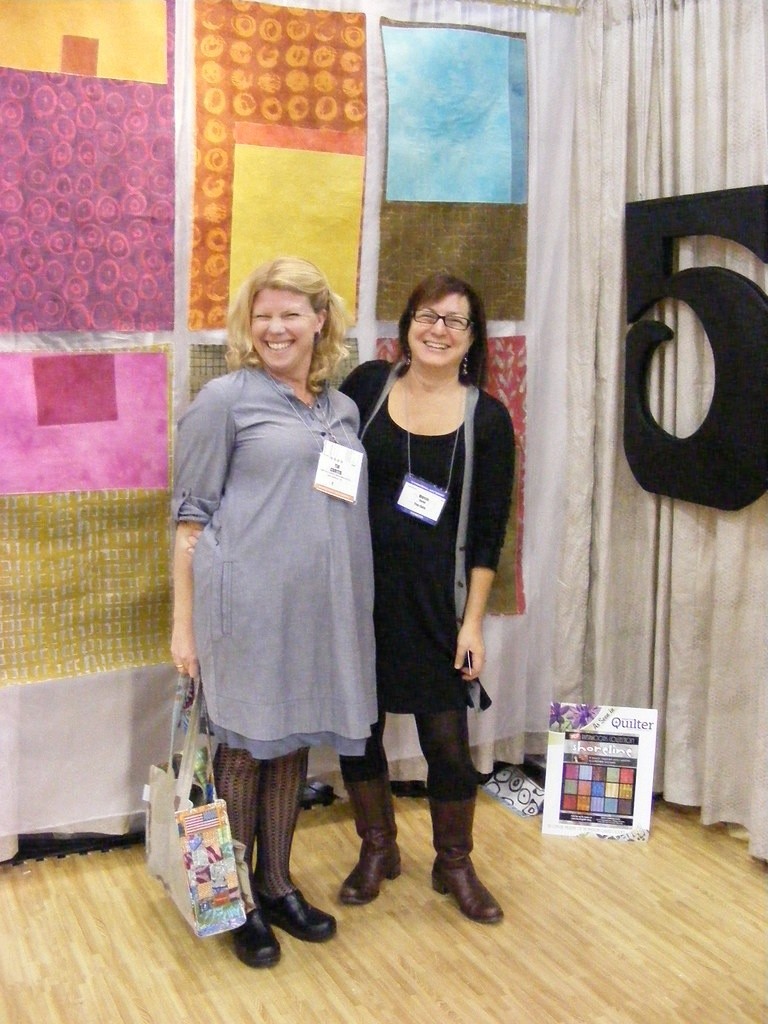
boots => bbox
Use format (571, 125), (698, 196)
(429, 794), (504, 925)
(340, 771), (401, 905)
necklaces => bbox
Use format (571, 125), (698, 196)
(300, 394), (339, 444)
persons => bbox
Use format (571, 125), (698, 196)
(168, 257), (379, 970)
(337, 274), (518, 923)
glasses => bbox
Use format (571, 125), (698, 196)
(409, 309), (474, 331)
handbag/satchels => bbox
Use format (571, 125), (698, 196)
(145, 670), (246, 937)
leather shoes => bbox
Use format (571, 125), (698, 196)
(256, 890), (336, 942)
(231, 905), (280, 968)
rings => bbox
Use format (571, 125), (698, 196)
(175, 665), (183, 668)
